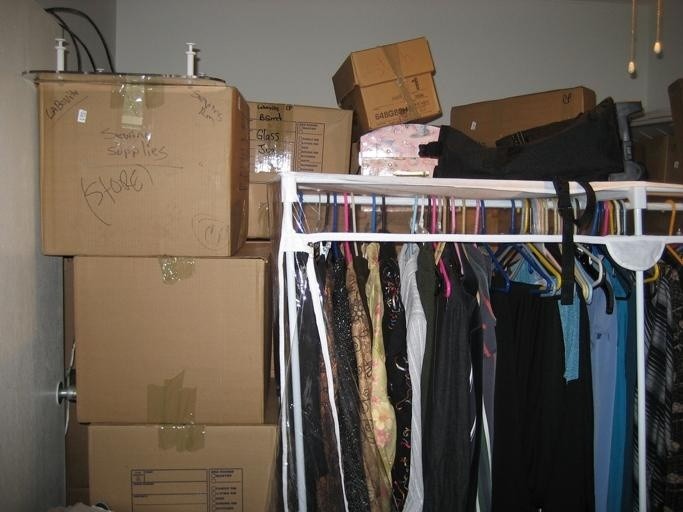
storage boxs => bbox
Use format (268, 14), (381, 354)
(451, 86), (596, 147)
(632, 138), (683, 183)
